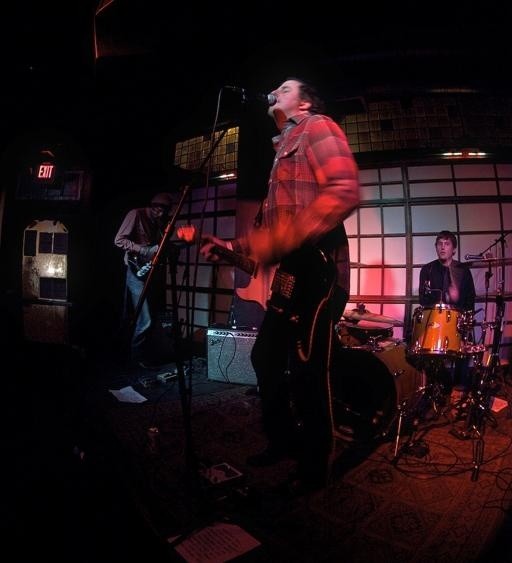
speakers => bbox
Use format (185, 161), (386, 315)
(207, 328), (258, 387)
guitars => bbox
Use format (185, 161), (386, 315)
(176, 224), (336, 364)
(127, 241), (194, 281)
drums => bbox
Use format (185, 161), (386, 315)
(328, 335), (426, 443)
(405, 303), (473, 369)
(334, 321), (394, 347)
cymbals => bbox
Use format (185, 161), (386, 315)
(342, 310), (405, 328)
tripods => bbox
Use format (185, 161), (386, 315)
(125, 97), (288, 548)
(381, 229), (511, 481)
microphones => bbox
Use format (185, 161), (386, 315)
(231, 85), (278, 106)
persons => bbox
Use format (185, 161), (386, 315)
(200, 75), (361, 496)
(419, 231), (476, 312)
(113, 191), (174, 388)
